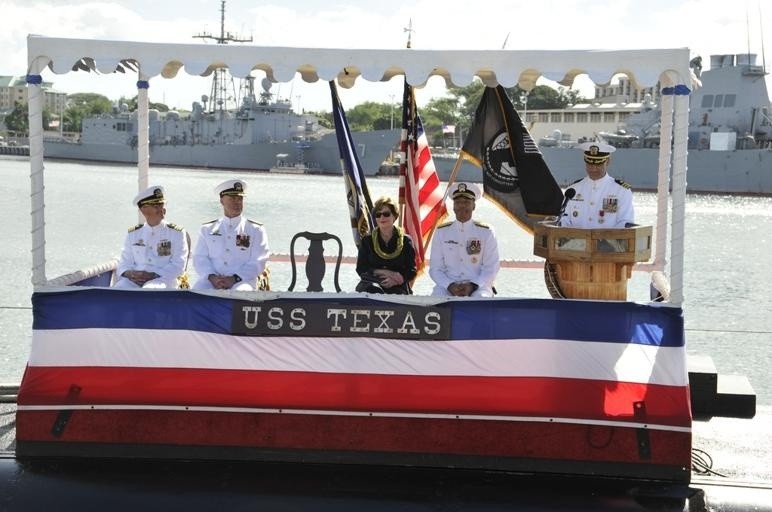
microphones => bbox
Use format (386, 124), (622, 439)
(556, 188), (576, 216)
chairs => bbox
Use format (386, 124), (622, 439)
(286, 230), (342, 294)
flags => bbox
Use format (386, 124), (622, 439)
(461, 85), (564, 235)
(398, 75), (448, 273)
(330, 80), (377, 249)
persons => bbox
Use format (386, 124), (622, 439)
(355, 196), (417, 294)
(428, 181), (500, 298)
(110, 185), (189, 289)
(558, 142), (635, 228)
(190, 178), (269, 291)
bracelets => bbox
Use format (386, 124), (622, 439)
(153, 272), (155, 279)
(470, 282), (478, 290)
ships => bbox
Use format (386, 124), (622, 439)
(43, 0), (403, 181)
(420, 58), (772, 198)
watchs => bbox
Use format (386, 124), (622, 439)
(234, 274), (241, 282)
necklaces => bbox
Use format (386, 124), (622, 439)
(371, 225), (404, 259)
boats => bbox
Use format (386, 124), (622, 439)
(266, 152), (310, 174)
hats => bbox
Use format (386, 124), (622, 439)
(213, 177), (247, 198)
(447, 181), (481, 200)
(132, 184), (168, 208)
(581, 142), (617, 164)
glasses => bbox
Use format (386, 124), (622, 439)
(375, 210), (392, 218)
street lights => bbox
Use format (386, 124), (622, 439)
(388, 92), (397, 129)
